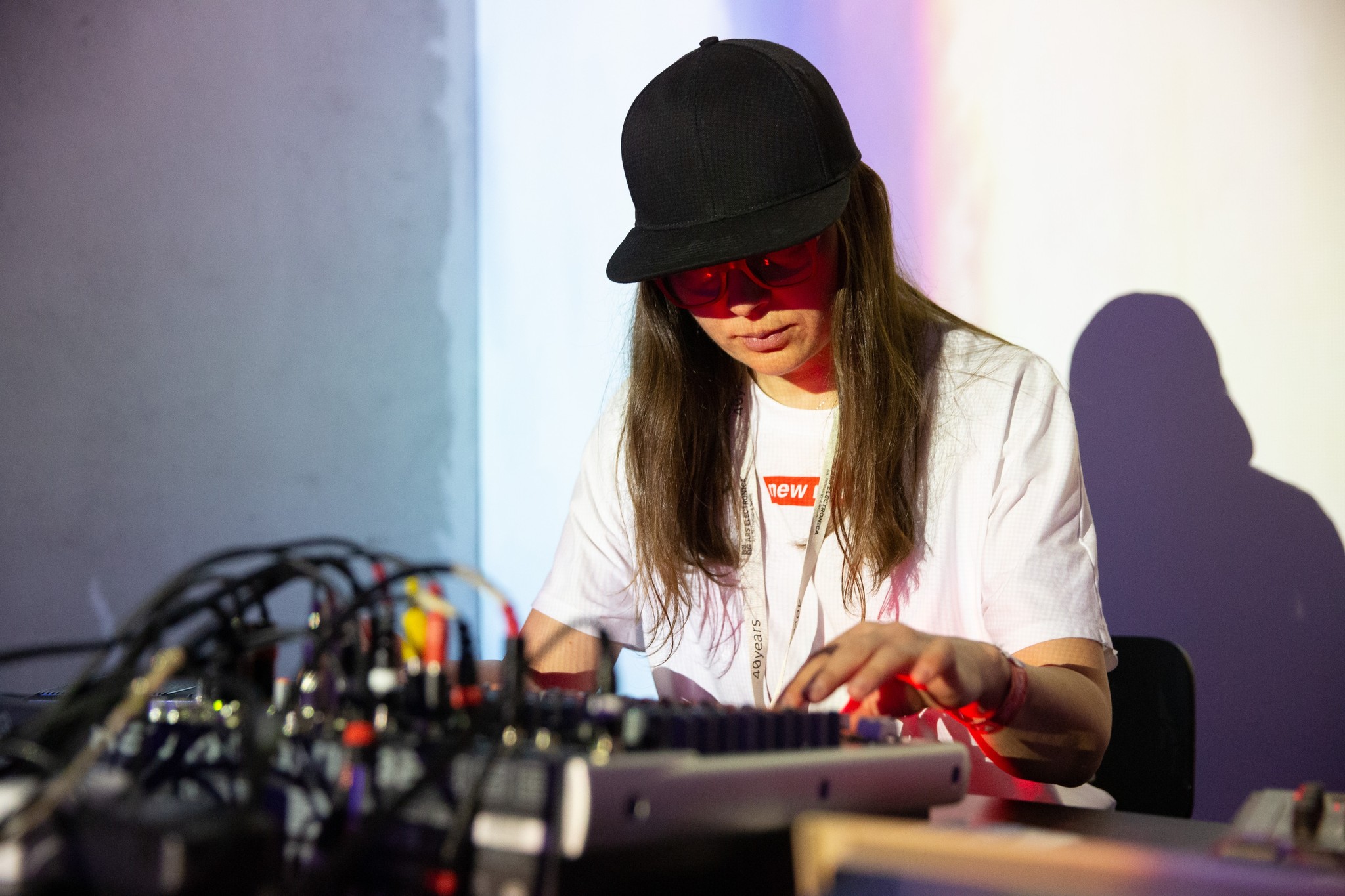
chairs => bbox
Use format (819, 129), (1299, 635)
(1093, 636), (1195, 818)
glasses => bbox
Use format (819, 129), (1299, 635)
(657, 235), (820, 308)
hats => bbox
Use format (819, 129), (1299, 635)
(606, 35), (862, 282)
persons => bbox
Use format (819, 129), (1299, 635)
(523, 31), (1117, 791)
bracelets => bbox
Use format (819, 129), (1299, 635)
(977, 656), (1029, 735)
